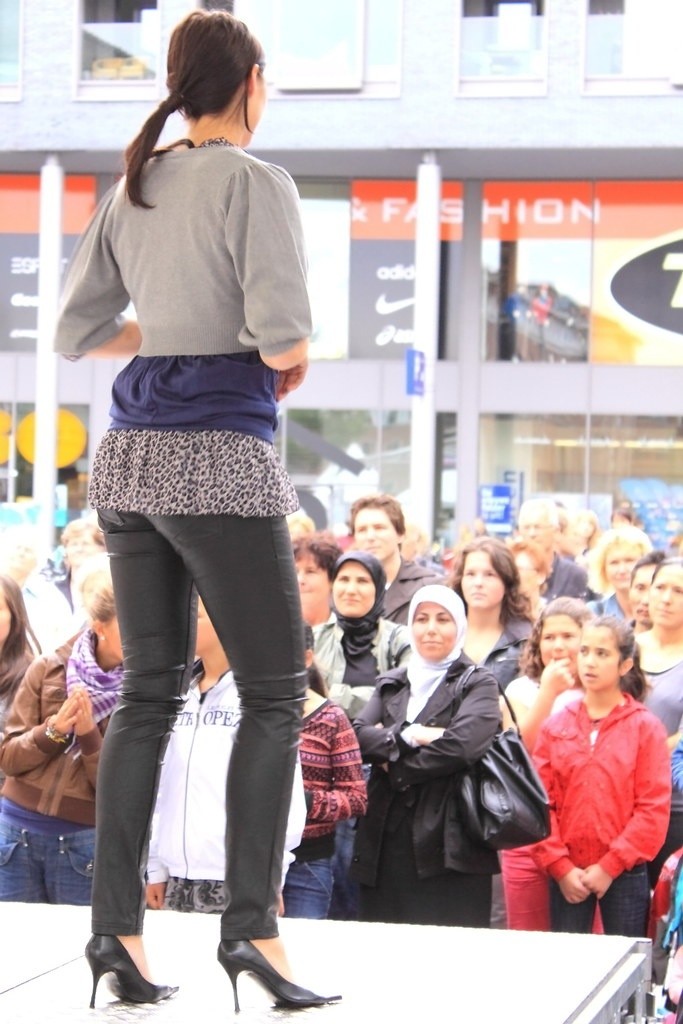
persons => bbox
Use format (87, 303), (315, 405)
(0, 490), (683, 944)
(52, 9), (342, 1012)
(0, 573), (123, 905)
(145, 594), (308, 917)
(528, 613), (673, 1024)
(351, 584), (504, 928)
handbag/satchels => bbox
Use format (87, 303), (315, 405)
(454, 665), (551, 850)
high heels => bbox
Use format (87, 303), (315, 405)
(85, 934), (180, 1007)
(218, 940), (342, 1013)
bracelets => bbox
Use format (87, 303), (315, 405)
(45, 720), (66, 744)
